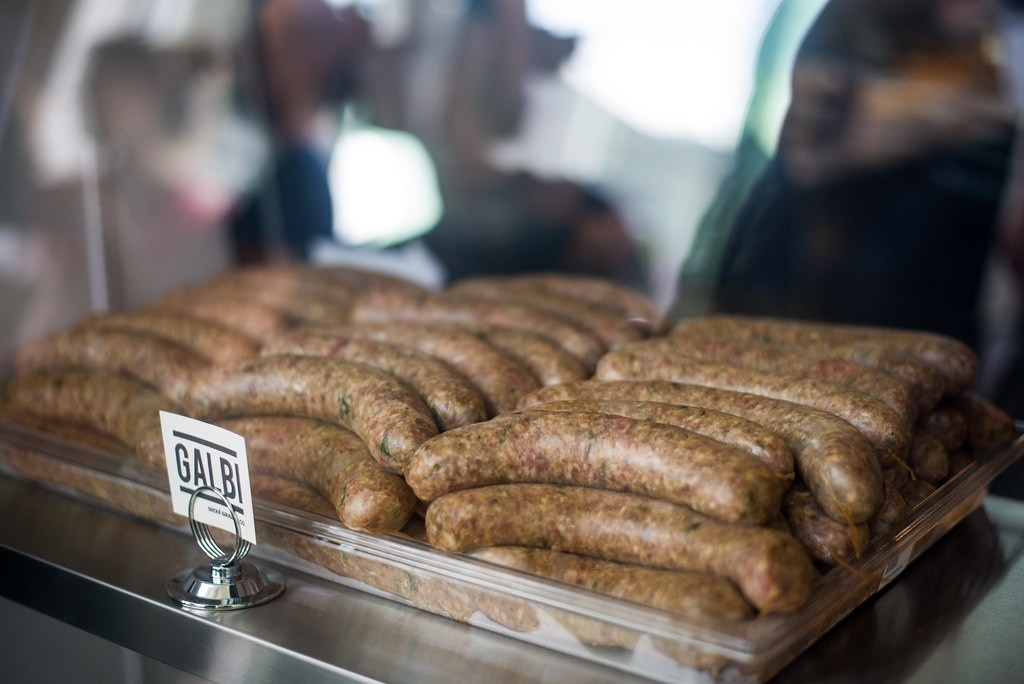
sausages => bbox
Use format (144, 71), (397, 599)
(0, 262), (1016, 674)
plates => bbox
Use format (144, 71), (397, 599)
(0, 418), (994, 683)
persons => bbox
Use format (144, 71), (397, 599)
(0, 1), (1024, 353)
